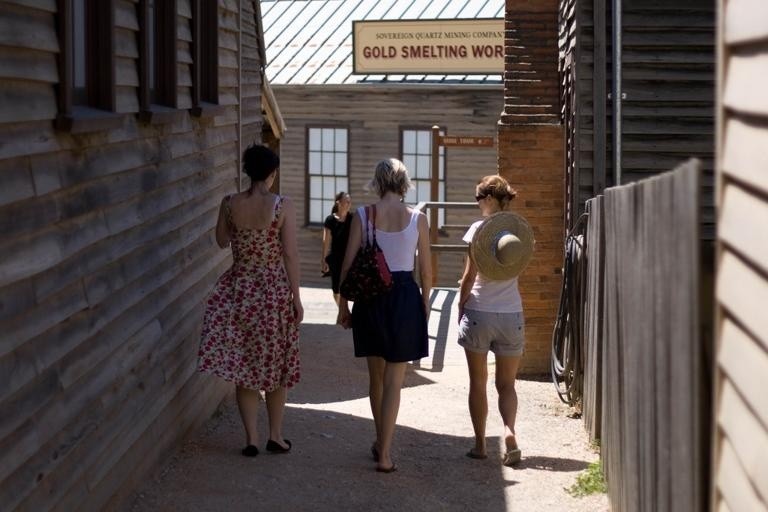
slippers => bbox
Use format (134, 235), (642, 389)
(376, 462), (398, 473)
(504, 449), (521, 465)
(466, 448), (487, 459)
(371, 445), (379, 461)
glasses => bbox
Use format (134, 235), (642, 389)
(475, 194), (487, 201)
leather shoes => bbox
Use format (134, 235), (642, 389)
(266, 439), (291, 452)
(242, 444), (259, 456)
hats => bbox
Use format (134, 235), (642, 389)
(470, 212), (534, 281)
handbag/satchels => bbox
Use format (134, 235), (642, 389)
(340, 242), (395, 301)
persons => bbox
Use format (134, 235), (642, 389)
(198, 144), (304, 457)
(320, 191), (355, 325)
(336, 158), (434, 473)
(458, 176), (526, 465)
